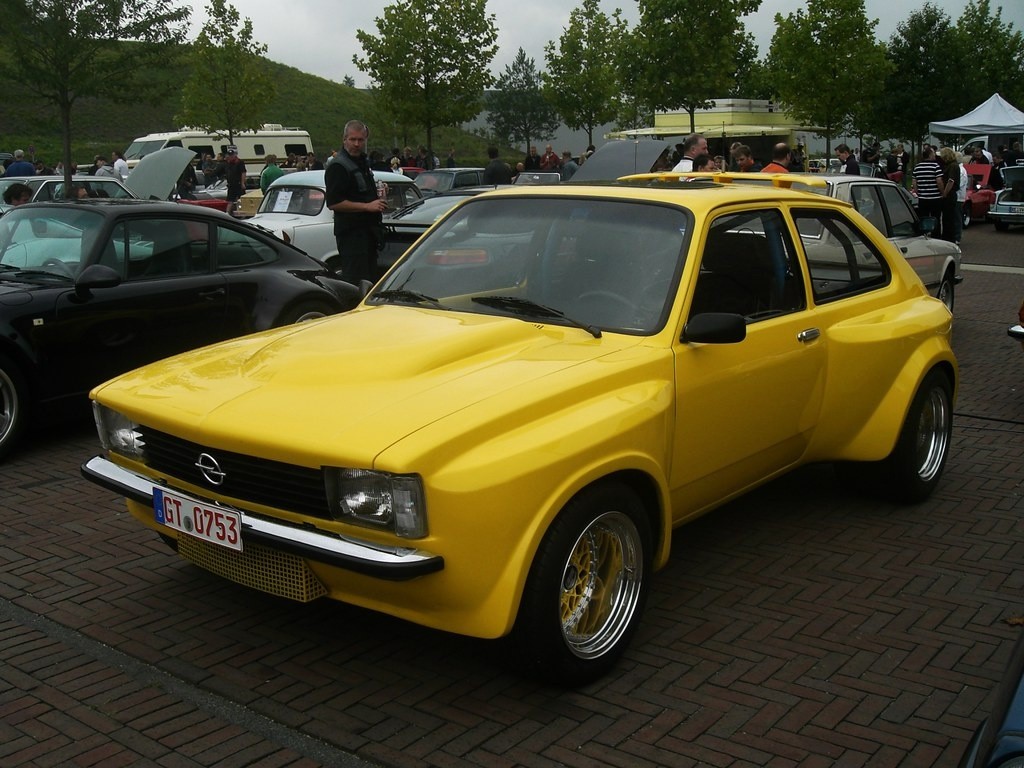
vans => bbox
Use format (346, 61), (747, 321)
(121, 124), (315, 190)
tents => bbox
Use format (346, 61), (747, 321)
(928, 94), (1024, 153)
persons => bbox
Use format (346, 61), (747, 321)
(653, 133), (807, 181)
(834, 142), (909, 189)
(482, 144), (595, 185)
(325, 119), (391, 289)
(0, 143), (341, 216)
(912, 140), (1024, 246)
(362, 145), (456, 196)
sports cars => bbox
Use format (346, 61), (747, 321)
(77, 177), (962, 686)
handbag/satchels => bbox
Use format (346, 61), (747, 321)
(370, 222), (389, 252)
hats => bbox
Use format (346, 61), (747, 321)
(14, 149), (25, 158)
(798, 142), (804, 146)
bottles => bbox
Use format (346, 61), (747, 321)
(376, 179), (386, 201)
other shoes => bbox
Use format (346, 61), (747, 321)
(955, 241), (961, 245)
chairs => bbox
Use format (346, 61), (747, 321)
(151, 221), (195, 276)
(79, 226), (119, 273)
(687, 232), (777, 318)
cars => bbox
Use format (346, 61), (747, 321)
(406, 167), (487, 203)
(233, 166), (434, 274)
(675, 173), (966, 322)
(0, 174), (143, 224)
(368, 181), (555, 295)
(825, 162), (889, 180)
(0, 200), (380, 462)
(985, 157), (1024, 230)
